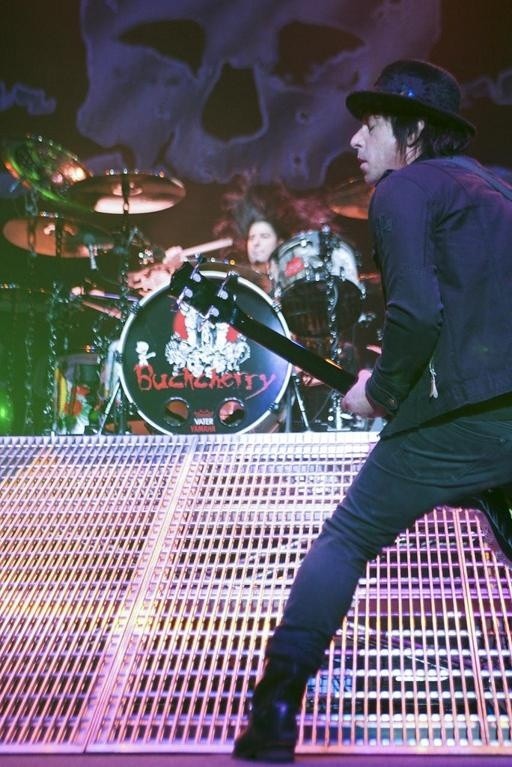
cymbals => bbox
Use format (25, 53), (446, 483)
(1, 215), (116, 257)
(1, 135), (91, 205)
(67, 171), (185, 216)
(196, 261), (272, 293)
(328, 179), (375, 219)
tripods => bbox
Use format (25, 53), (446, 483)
(97, 218), (155, 435)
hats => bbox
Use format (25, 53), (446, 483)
(345, 58), (479, 138)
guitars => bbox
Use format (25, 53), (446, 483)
(165, 264), (510, 558)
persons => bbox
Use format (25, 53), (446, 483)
(232, 58), (512, 764)
(165, 215), (360, 377)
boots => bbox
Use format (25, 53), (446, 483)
(230, 655), (315, 764)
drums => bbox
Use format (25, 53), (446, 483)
(118, 270), (296, 435)
(267, 231), (367, 339)
